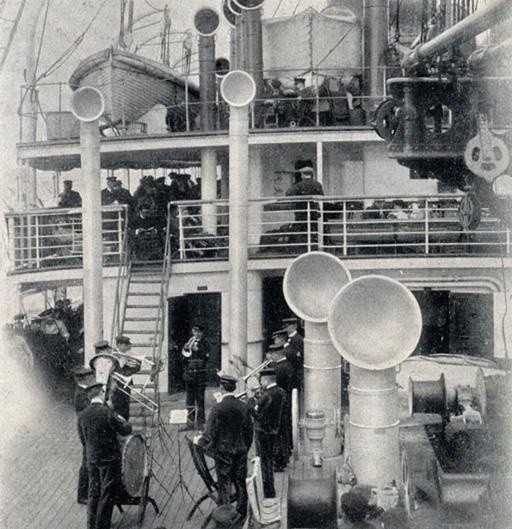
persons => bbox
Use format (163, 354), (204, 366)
(286, 167), (324, 241)
(1, 298), (303, 528)
(339, 494), (376, 529)
(365, 199), (409, 220)
(58, 172), (200, 258)
(260, 78), (313, 126)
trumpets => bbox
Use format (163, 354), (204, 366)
(112, 350), (164, 374)
(181, 334), (199, 359)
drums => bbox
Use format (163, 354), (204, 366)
(110, 432), (152, 504)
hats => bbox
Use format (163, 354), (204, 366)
(189, 318), (207, 329)
(68, 335), (131, 393)
(299, 166), (313, 173)
(219, 374), (239, 386)
(107, 175), (117, 181)
(295, 77), (304, 86)
(259, 317), (297, 375)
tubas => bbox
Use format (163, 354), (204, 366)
(89, 353), (128, 425)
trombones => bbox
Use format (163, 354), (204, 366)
(241, 358), (272, 384)
(110, 371), (160, 413)
